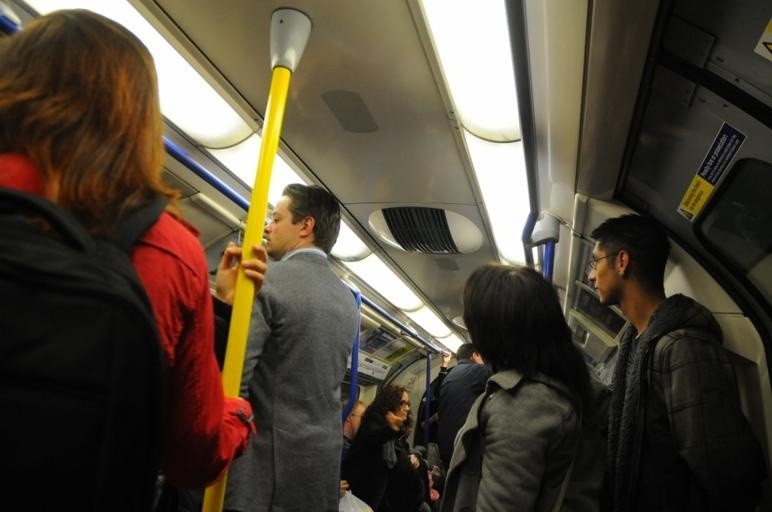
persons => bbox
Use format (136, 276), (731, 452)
(209, 183), (772, 512)
(0, 8), (255, 512)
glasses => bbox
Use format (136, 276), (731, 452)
(398, 400), (410, 408)
(588, 254), (617, 269)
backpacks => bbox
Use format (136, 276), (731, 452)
(0, 188), (166, 511)
